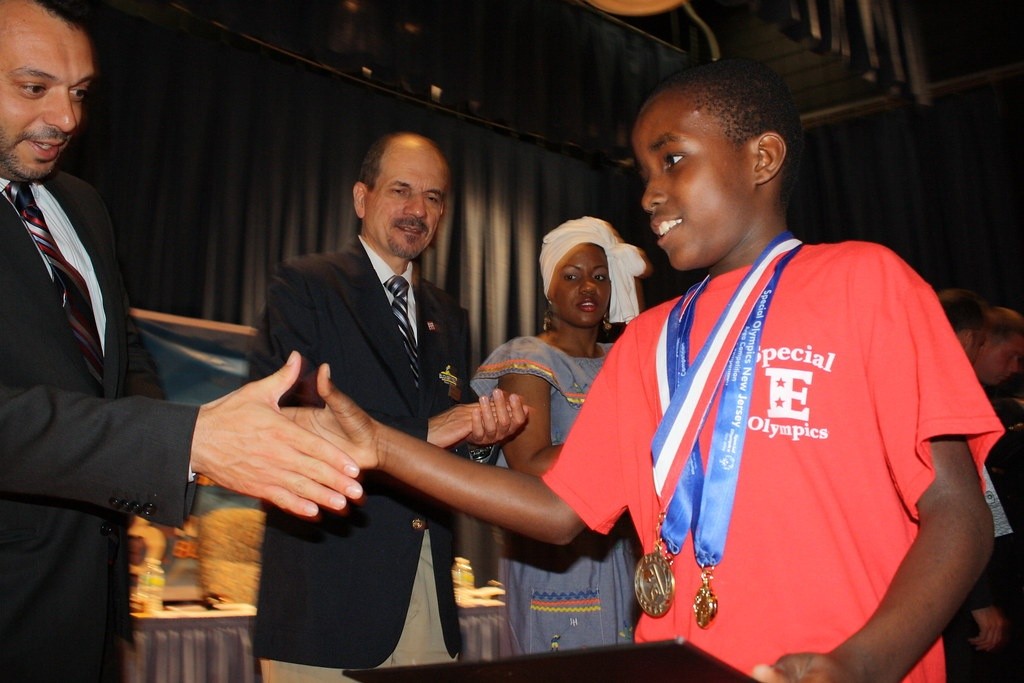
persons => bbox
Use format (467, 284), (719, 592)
(280, 56), (1006, 683)
(252, 131), (528, 683)
(933, 288), (1024, 653)
(469, 217), (658, 659)
(0, 0), (363, 683)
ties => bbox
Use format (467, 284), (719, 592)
(5, 177), (103, 386)
(384, 275), (418, 390)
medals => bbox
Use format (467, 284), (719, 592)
(632, 514), (677, 619)
(688, 567), (720, 630)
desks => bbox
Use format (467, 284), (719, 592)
(126, 597), (510, 683)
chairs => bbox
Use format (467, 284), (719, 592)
(198, 507), (265, 608)
